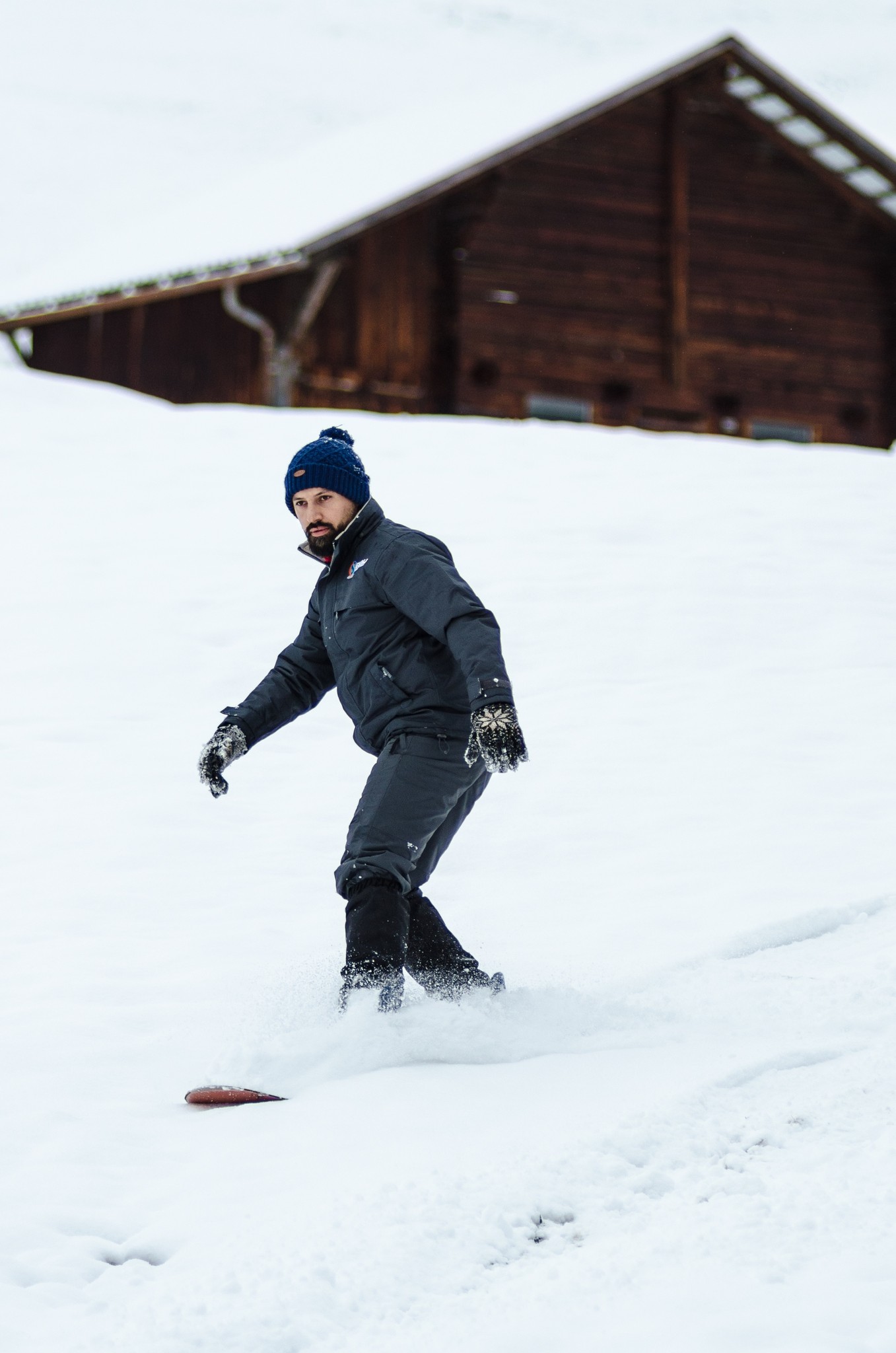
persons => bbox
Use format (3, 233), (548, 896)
(198, 425), (530, 1022)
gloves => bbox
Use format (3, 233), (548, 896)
(199, 726), (248, 798)
(463, 702), (528, 772)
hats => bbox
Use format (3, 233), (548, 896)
(285, 427), (371, 517)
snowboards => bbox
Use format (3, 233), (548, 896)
(186, 1086), (287, 1105)
